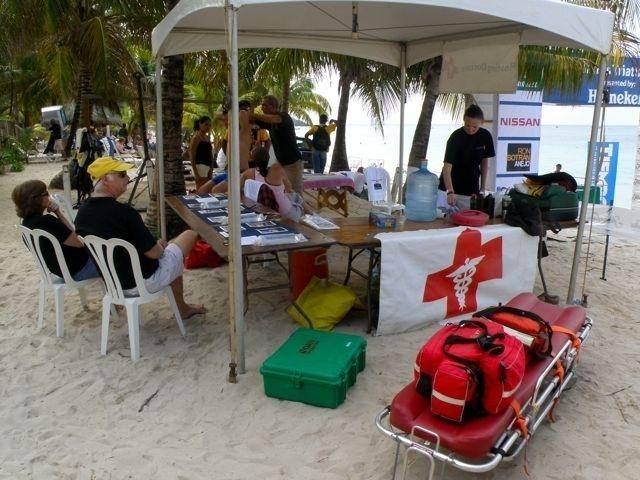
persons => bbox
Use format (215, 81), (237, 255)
(88, 121), (97, 156)
(555, 164), (561, 172)
(238, 99), (271, 168)
(198, 111), (251, 194)
(437, 104), (496, 208)
(240, 147), (313, 221)
(119, 123), (128, 149)
(131, 124), (141, 149)
(75, 156), (207, 320)
(11, 180), (123, 315)
(249, 95), (303, 198)
(305, 114), (338, 174)
(114, 136), (139, 158)
(43, 119), (63, 153)
(190, 116), (214, 194)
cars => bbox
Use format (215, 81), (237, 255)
(296, 136), (313, 170)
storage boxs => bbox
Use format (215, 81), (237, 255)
(506, 188), (579, 223)
(260, 326), (367, 409)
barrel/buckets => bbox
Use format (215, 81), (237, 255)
(405, 160), (439, 222)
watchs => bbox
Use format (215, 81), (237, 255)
(446, 191), (453, 195)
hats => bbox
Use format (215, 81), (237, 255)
(86, 156), (135, 178)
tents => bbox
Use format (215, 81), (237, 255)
(151, 1), (612, 374)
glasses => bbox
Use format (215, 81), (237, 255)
(108, 171), (128, 177)
(42, 190), (50, 197)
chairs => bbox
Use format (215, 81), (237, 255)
(363, 166), (406, 216)
(13, 224), (118, 337)
(100, 135), (157, 171)
(76, 235), (186, 365)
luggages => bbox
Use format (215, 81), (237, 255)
(512, 189), (579, 221)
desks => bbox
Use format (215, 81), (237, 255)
(164, 193), (338, 351)
(302, 173), (356, 217)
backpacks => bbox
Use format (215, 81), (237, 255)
(313, 125), (330, 150)
